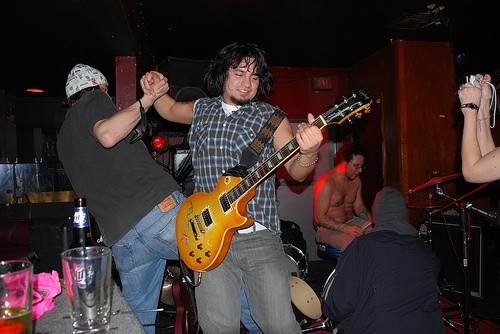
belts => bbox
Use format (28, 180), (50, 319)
(238, 221), (268, 234)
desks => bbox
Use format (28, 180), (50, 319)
(31, 277), (148, 334)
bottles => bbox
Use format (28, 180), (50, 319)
(70, 198), (96, 248)
(16, 186), (23, 205)
(6, 189), (13, 204)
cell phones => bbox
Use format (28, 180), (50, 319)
(466, 75), (476, 85)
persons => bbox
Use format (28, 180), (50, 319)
(321, 187), (447, 334)
(140, 42), (323, 334)
(313, 142), (374, 252)
(56, 64), (264, 334)
(457, 74), (500, 183)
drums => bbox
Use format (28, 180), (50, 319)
(282, 244), (309, 280)
(290, 276), (322, 329)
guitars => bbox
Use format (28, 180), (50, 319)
(175, 89), (373, 274)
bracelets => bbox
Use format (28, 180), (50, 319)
(297, 155), (318, 168)
(477, 115), (491, 121)
(461, 103), (478, 110)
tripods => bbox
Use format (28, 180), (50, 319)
(425, 186), (500, 334)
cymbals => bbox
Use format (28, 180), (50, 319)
(409, 173), (463, 194)
(159, 266), (181, 306)
(408, 198), (454, 209)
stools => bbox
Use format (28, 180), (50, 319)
(317, 244), (343, 293)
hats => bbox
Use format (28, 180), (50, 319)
(65, 64), (108, 98)
(371, 187), (410, 224)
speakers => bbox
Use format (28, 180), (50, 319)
(425, 220), (500, 301)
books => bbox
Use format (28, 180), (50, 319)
(345, 216), (372, 231)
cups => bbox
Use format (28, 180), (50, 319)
(0, 260), (34, 334)
(61, 246), (112, 334)
